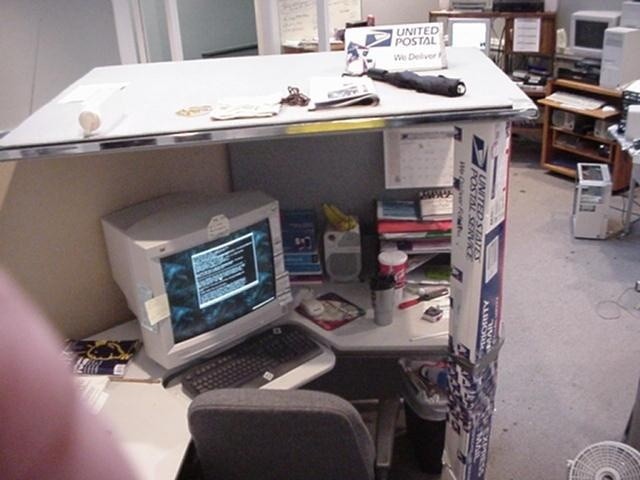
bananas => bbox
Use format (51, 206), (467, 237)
(322, 203), (355, 231)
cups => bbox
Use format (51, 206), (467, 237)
(370, 251), (408, 326)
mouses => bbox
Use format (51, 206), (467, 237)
(602, 105), (615, 112)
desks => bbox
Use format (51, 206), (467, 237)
(61, 278), (450, 479)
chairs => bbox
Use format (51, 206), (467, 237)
(186, 372), (406, 477)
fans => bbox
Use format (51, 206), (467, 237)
(567, 441), (639, 480)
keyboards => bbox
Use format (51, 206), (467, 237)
(179, 322), (324, 398)
(545, 88), (608, 111)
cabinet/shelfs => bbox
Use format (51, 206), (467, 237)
(428, 10), (557, 135)
(537, 76), (632, 193)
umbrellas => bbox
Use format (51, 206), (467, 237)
(342, 65), (467, 99)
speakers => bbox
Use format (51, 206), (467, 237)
(322, 220), (364, 283)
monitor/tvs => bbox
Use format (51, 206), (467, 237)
(100, 188), (291, 372)
(569, 9), (621, 65)
(447, 17), (491, 57)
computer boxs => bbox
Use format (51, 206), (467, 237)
(569, 162), (613, 240)
(599, 26), (640, 90)
(616, 80), (640, 134)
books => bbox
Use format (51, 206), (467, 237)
(71, 371), (110, 414)
(57, 336), (143, 376)
(375, 196), (450, 284)
